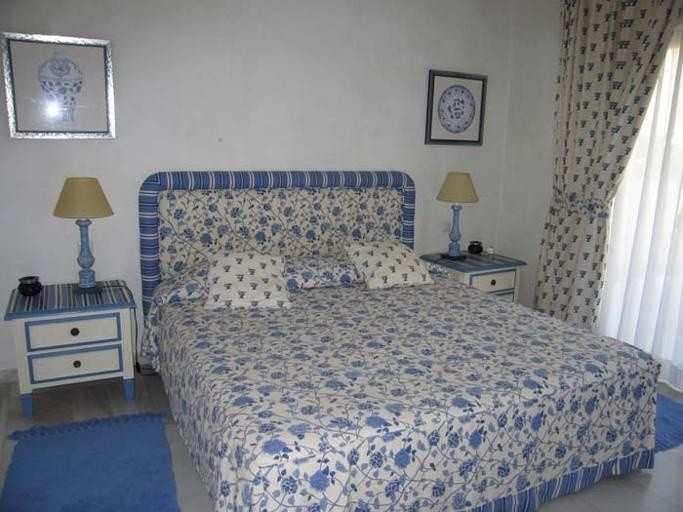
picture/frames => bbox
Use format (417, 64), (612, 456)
(2, 32), (116, 139)
(424, 69), (488, 146)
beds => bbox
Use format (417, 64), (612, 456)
(137, 172), (660, 512)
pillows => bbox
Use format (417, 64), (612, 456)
(341, 238), (434, 287)
(200, 251), (293, 311)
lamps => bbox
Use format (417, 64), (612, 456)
(52, 177), (112, 292)
(437, 172), (478, 257)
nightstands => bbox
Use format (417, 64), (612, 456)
(420, 252), (526, 316)
(5, 280), (135, 418)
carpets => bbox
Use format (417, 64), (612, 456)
(1, 412), (180, 512)
(656, 394), (683, 451)
